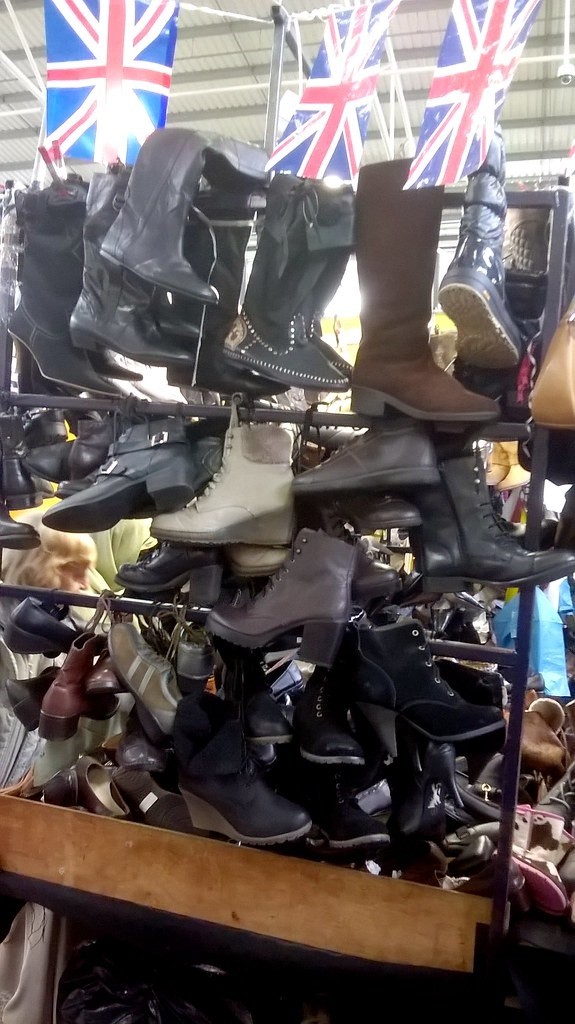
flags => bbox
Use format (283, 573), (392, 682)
(401, 0), (542, 191)
(265, 1), (400, 181)
(45, 0), (178, 169)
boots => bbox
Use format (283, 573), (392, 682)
(0, 125), (573, 435)
(0, 403), (575, 935)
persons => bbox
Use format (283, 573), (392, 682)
(0, 511), (97, 1024)
